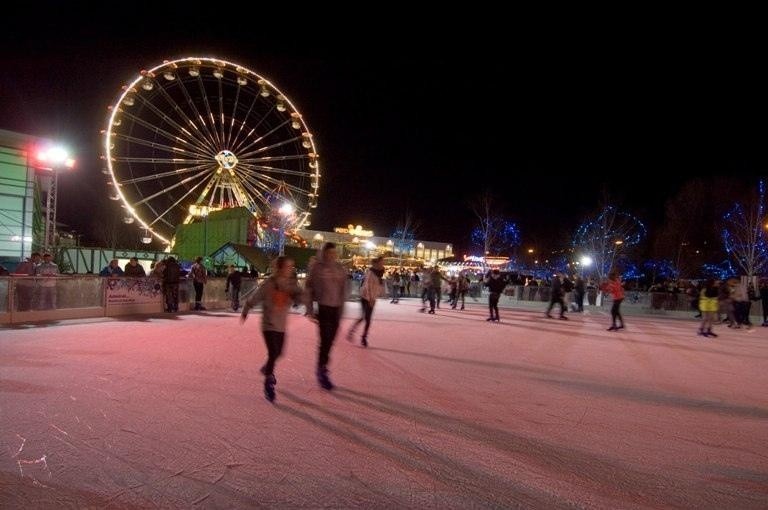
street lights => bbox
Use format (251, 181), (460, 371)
(278, 203), (294, 256)
(30, 139), (75, 254)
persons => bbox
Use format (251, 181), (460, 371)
(505, 270), (600, 320)
(160, 256), (182, 313)
(35, 254), (61, 311)
(344, 255), (385, 349)
(225, 263), (243, 312)
(249, 263), (258, 278)
(240, 265), (249, 276)
(124, 256), (146, 277)
(240, 254), (305, 404)
(352, 263), (368, 280)
(188, 255), (209, 311)
(482, 267), (506, 324)
(302, 240), (350, 392)
(100, 257), (123, 276)
(14, 250), (40, 310)
(646, 278), (768, 338)
(386, 265), (471, 315)
(599, 270), (627, 332)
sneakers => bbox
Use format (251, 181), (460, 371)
(265, 375), (276, 393)
(317, 369), (327, 383)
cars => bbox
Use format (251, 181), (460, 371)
(349, 268), (365, 281)
